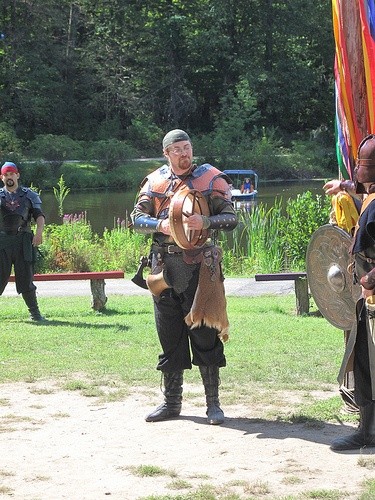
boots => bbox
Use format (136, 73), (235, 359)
(22, 291), (48, 321)
(144, 370), (185, 422)
(330, 404), (375, 453)
(198, 365), (225, 424)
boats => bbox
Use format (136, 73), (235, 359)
(222, 169), (259, 212)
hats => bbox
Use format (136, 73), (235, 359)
(1, 162), (18, 175)
(163, 129), (190, 148)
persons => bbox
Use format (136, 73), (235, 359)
(240, 177), (253, 194)
(0, 161), (46, 321)
(322, 134), (375, 450)
(130, 129), (238, 424)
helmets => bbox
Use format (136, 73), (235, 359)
(355, 133), (375, 195)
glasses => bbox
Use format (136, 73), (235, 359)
(168, 146), (192, 155)
